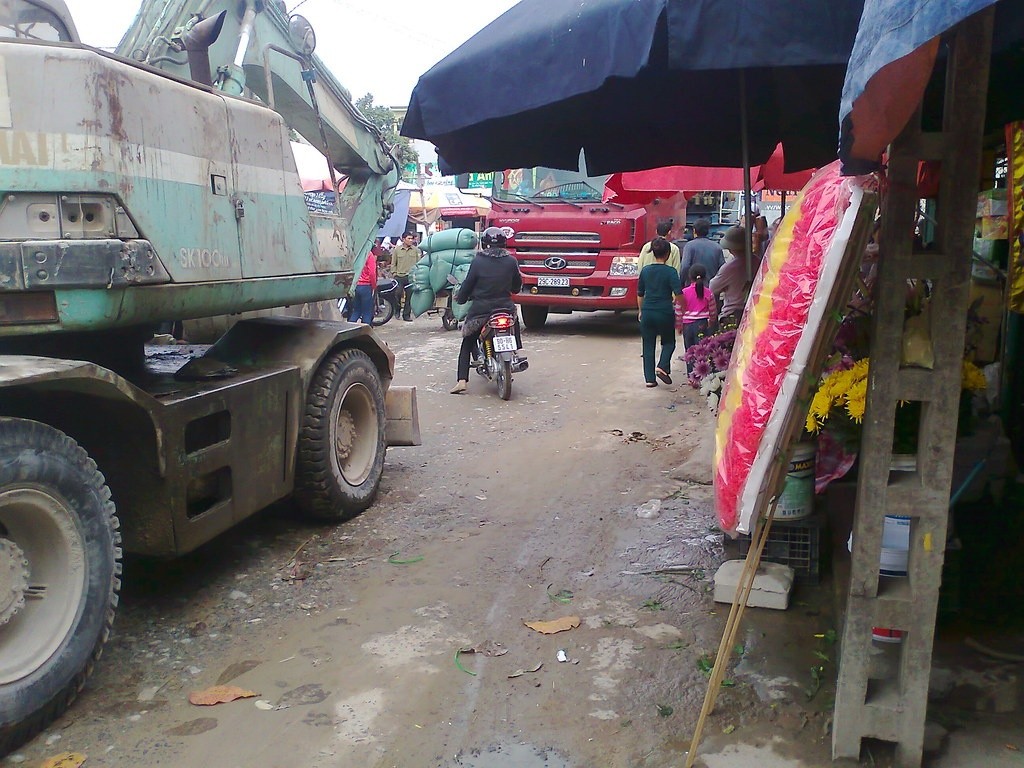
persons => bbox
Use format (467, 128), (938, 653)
(449, 226), (527, 394)
(637, 196), (770, 386)
(349, 230), (422, 325)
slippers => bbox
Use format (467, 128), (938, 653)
(646, 381), (658, 387)
(656, 372), (672, 385)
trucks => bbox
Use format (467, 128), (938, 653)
(453, 166), (744, 330)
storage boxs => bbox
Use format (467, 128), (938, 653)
(723, 514), (827, 587)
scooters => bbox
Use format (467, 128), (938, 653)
(371, 279), (399, 327)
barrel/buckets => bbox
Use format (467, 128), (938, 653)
(847, 515), (911, 644)
(763, 442), (817, 521)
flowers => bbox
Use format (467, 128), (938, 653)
(676, 324), (738, 415)
(806, 358), (986, 433)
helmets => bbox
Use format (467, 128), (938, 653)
(481, 227), (507, 248)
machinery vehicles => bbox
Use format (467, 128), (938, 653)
(0, 1), (437, 752)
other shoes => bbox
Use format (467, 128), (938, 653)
(450, 382), (468, 393)
(395, 314), (413, 322)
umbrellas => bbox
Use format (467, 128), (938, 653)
(398, 0), (867, 291)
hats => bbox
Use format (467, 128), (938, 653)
(719, 225), (746, 249)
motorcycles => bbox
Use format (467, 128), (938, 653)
(447, 274), (530, 401)
(435, 285), (461, 331)
